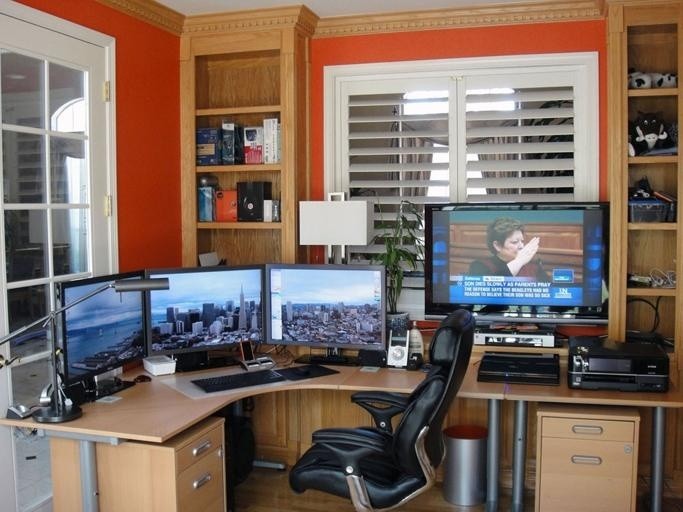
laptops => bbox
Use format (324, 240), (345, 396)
(478, 350), (560, 379)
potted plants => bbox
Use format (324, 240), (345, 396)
(367, 187), (426, 336)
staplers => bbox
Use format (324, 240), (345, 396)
(631, 276), (653, 288)
(40, 383), (54, 403)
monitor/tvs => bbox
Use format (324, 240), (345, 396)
(423, 201), (610, 328)
(265, 262), (386, 369)
(53, 268), (147, 405)
(144, 263), (265, 373)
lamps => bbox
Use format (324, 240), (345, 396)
(1, 277), (169, 424)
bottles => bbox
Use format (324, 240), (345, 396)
(408, 320), (424, 359)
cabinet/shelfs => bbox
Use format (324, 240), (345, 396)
(50, 418), (226, 512)
(606, 5), (682, 365)
(179, 28), (295, 269)
(535, 411), (641, 512)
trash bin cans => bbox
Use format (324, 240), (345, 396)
(443, 425), (487, 507)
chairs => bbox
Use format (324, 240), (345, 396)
(287, 309), (475, 512)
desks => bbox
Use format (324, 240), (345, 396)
(1, 335), (683, 512)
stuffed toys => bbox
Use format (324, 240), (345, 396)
(628, 65), (676, 89)
(628, 110), (676, 157)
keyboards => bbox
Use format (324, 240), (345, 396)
(190, 368), (283, 394)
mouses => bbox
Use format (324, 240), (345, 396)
(292, 367), (310, 376)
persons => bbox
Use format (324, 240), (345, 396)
(468, 215), (543, 277)
